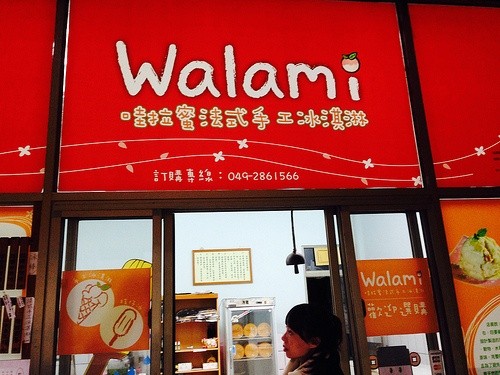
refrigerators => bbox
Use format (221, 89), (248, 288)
(222, 296), (279, 375)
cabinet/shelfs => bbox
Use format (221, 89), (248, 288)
(174, 292), (221, 375)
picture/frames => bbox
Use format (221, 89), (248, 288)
(192, 248), (253, 285)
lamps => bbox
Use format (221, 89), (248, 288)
(287, 211), (306, 275)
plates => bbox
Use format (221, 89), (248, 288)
(449, 234), (500, 291)
(232, 322), (272, 360)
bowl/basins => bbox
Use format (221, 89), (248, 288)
(203, 362), (217, 370)
(178, 362), (192, 372)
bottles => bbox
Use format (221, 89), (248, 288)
(175, 341), (180, 351)
(127, 362), (136, 375)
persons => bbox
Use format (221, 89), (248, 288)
(282, 304), (343, 375)
